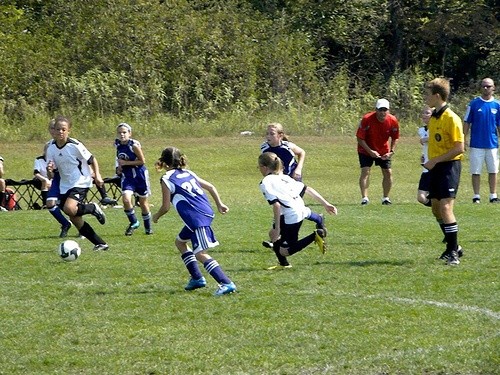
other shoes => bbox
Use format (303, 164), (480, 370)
(315, 213), (326, 229)
(360, 196), (369, 205)
(89, 202), (106, 225)
(144, 226), (154, 235)
(124, 220), (140, 236)
(472, 197), (480, 204)
(60, 222), (71, 238)
(263, 240), (277, 250)
(91, 241), (109, 253)
(101, 197), (118, 206)
(434, 244), (464, 261)
(381, 197), (392, 205)
(489, 197), (500, 203)
(445, 250), (460, 266)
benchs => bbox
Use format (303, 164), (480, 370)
(4, 178), (123, 211)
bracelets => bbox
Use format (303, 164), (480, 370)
(390, 150), (394, 154)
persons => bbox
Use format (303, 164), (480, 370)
(261, 122), (324, 251)
(113, 123), (153, 236)
(0, 156), (8, 211)
(423, 78), (464, 266)
(153, 147), (238, 295)
(45, 118), (85, 239)
(463, 77), (500, 203)
(356, 99), (400, 205)
(45, 116), (117, 251)
(259, 152), (337, 270)
(34, 154), (86, 239)
(418, 105), (434, 206)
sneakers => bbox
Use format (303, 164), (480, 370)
(267, 262), (293, 272)
(184, 276), (207, 290)
(211, 281), (237, 298)
(313, 228), (328, 255)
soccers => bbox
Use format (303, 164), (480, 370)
(59, 240), (81, 261)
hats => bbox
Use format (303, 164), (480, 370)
(375, 99), (390, 110)
